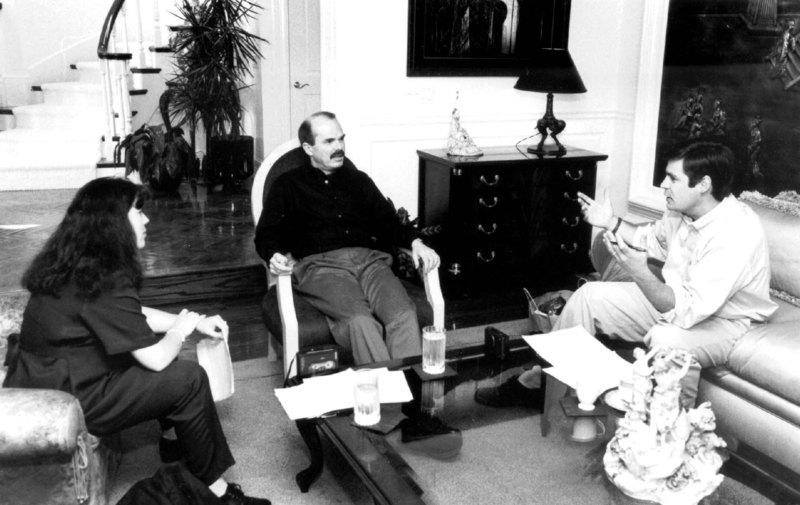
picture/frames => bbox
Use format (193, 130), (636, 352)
(406, 0), (572, 77)
(626, 0), (800, 221)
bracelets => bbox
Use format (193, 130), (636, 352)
(611, 217), (623, 236)
(164, 327), (189, 342)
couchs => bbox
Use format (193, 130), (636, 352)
(575, 191), (800, 505)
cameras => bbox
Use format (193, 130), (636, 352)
(484, 326), (510, 361)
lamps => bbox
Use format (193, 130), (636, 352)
(513, 48), (588, 156)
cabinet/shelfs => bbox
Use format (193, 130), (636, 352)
(415, 144), (609, 330)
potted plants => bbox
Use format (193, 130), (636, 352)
(114, 123), (200, 198)
(158, 0), (271, 184)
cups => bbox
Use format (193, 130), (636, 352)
(618, 377), (654, 405)
(419, 379), (445, 418)
(420, 324), (447, 375)
(350, 372), (382, 429)
(573, 377), (598, 411)
(570, 416), (607, 445)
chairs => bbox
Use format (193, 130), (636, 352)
(250, 138), (447, 379)
(0, 288), (127, 505)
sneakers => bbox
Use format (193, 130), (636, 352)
(474, 372), (545, 414)
(400, 410), (461, 444)
(217, 483), (271, 505)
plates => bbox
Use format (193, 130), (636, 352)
(602, 389), (657, 414)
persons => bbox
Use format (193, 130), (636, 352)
(253, 111), (464, 462)
(472, 139), (779, 412)
(2, 175), (273, 505)
